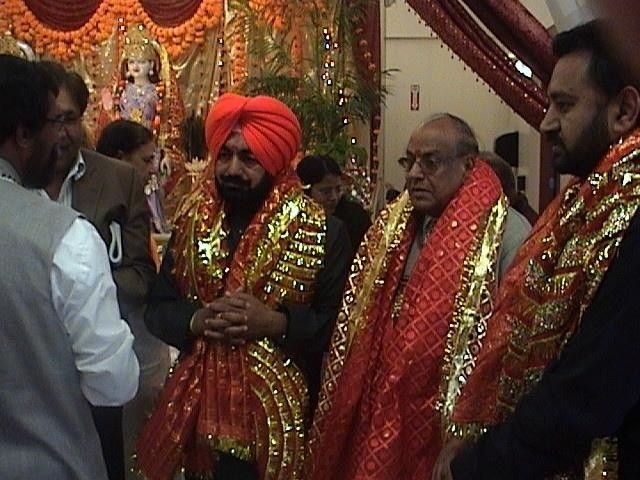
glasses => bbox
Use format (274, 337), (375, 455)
(397, 156), (441, 174)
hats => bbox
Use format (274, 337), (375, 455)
(206, 93), (301, 178)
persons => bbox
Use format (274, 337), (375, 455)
(448, 18), (640, 478)
(145, 94), (370, 480)
(0, 53), (170, 480)
(482, 150), (539, 226)
(304, 110), (532, 479)
